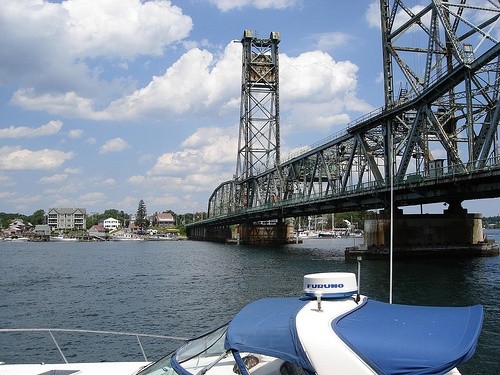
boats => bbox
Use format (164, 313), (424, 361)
(2, 271), (484, 375)
